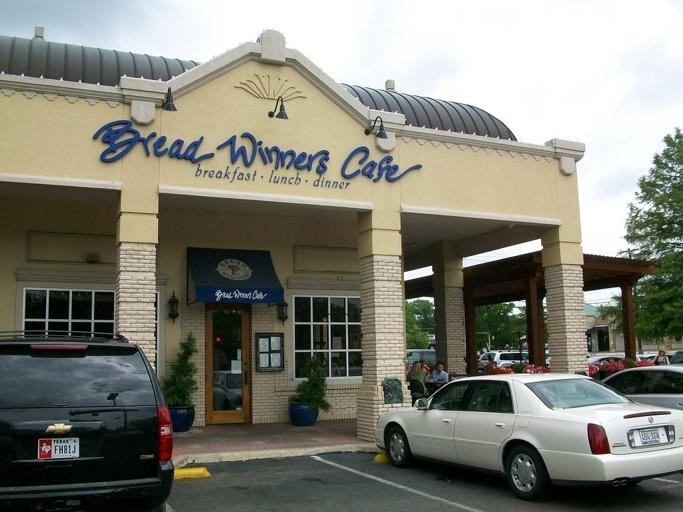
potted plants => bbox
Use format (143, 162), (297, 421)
(159, 331), (198, 432)
(288, 345), (334, 426)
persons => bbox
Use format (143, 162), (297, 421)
(652, 349), (670, 365)
(430, 361), (449, 385)
(406, 360), (429, 398)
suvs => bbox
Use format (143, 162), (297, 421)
(0, 329), (174, 511)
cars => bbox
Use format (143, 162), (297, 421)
(373, 373), (683, 502)
(597, 364), (683, 411)
(588, 349), (683, 366)
(213, 370), (242, 410)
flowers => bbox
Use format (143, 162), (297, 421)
(596, 360), (624, 372)
(589, 364), (600, 377)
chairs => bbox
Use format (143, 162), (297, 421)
(408, 380), (430, 406)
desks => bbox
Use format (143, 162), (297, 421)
(425, 381), (448, 397)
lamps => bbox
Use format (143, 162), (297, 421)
(156, 88), (177, 111)
(278, 299), (288, 326)
(168, 290), (179, 324)
(268, 96), (288, 119)
(364, 116), (388, 139)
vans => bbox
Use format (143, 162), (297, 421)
(477, 350), (529, 374)
(406, 348), (436, 374)
